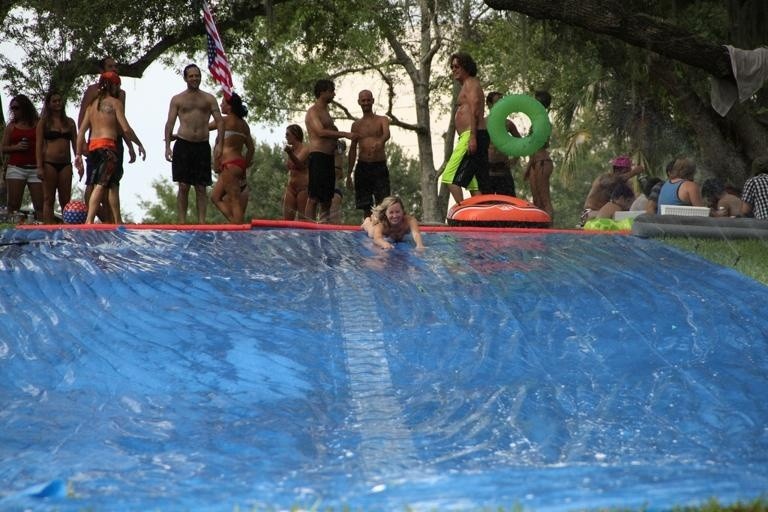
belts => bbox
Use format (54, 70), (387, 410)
(11, 164), (37, 169)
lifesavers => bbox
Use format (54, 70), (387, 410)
(447, 196), (552, 230)
(487, 95), (552, 157)
(584, 218), (632, 232)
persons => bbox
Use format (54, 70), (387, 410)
(596, 184), (633, 219)
(329, 141), (347, 224)
(741, 157), (768, 219)
(524, 90), (554, 228)
(165, 64), (225, 225)
(702, 178), (742, 218)
(362, 197), (429, 250)
(658, 153), (704, 215)
(283, 125), (308, 221)
(484, 91), (522, 197)
(36, 93), (77, 226)
(0, 94), (43, 225)
(630, 177), (661, 212)
(75, 71), (136, 224)
(346, 90), (390, 221)
(443, 53), (490, 204)
(647, 161), (676, 215)
(78, 58), (146, 223)
(581, 155), (643, 225)
(163, 91), (254, 224)
(304, 80), (364, 224)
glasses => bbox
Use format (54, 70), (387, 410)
(10, 106), (21, 110)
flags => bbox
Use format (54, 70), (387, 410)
(202, 1), (233, 104)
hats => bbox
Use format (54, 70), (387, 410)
(611, 157), (633, 167)
(99, 72), (121, 85)
